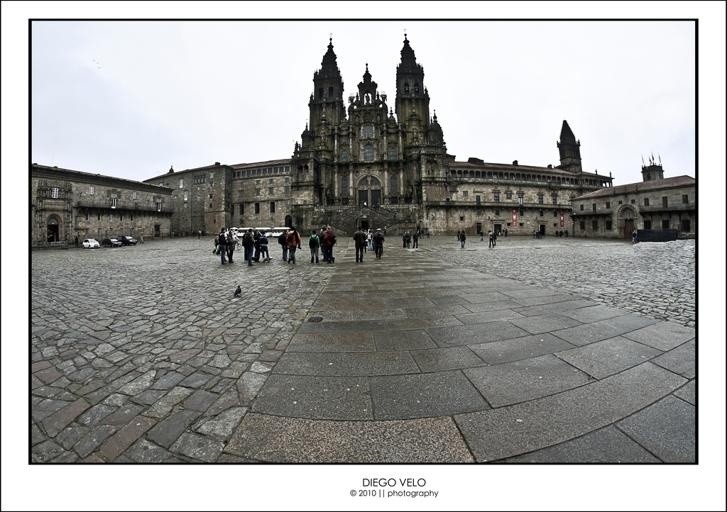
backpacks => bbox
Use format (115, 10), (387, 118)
(309, 235), (318, 248)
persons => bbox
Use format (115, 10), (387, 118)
(531, 228), (567, 240)
(210, 225), (498, 267)
(502, 227), (507, 237)
(631, 229), (636, 244)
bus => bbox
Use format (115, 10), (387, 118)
(224, 227), (290, 238)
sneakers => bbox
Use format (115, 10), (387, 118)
(221, 258), (366, 266)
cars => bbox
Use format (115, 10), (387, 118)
(102, 238), (122, 248)
(118, 235), (137, 246)
(82, 239), (100, 250)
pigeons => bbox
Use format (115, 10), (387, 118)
(234, 285), (241, 297)
